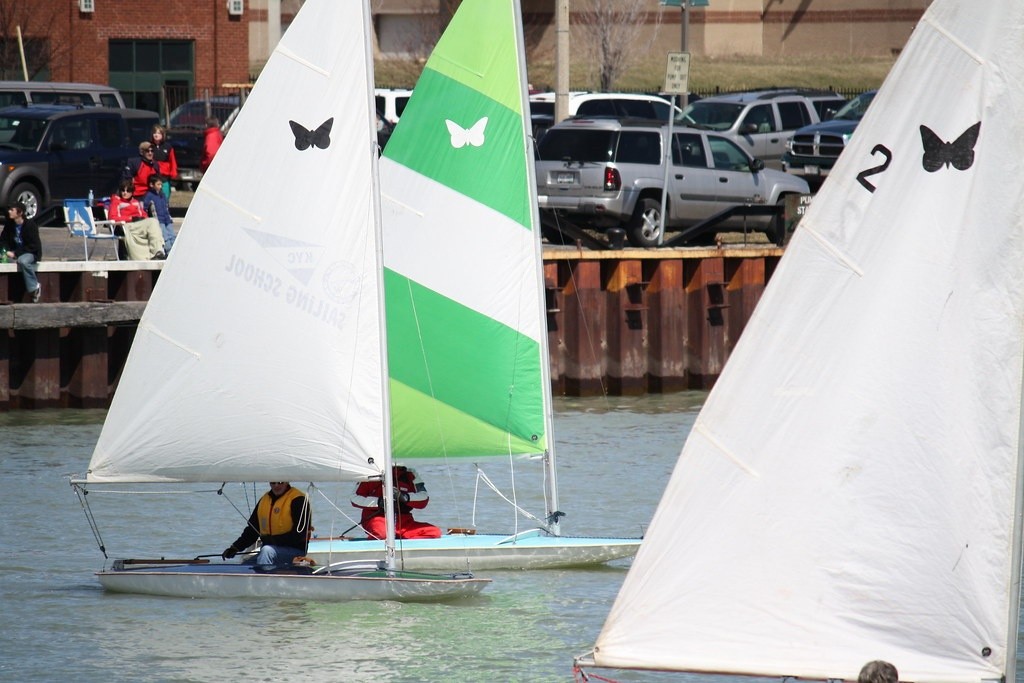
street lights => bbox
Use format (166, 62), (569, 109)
(659, 0), (709, 52)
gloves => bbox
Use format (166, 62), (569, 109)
(260, 534), (272, 545)
(222, 544), (237, 561)
(393, 489), (408, 503)
(378, 495), (384, 508)
(132, 216), (145, 222)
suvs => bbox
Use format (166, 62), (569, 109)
(528, 116), (812, 248)
(529, 89), (700, 154)
(667, 84), (852, 168)
(781, 87), (881, 188)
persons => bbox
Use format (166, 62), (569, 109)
(108, 180), (169, 260)
(126, 141), (157, 211)
(143, 174), (176, 256)
(200, 116), (224, 175)
(222, 479), (312, 572)
(349, 465), (441, 539)
(150, 124), (178, 223)
(0, 200), (42, 303)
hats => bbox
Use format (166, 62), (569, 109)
(138, 141), (155, 152)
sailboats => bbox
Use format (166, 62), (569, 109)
(63, 0), (494, 601)
(565, 0), (1022, 683)
(228, 0), (652, 570)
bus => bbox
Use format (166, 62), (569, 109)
(0, 101), (161, 225)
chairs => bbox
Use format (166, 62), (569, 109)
(64, 196), (148, 260)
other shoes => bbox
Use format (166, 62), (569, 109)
(31, 282), (41, 304)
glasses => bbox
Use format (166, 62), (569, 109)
(121, 189), (132, 193)
(144, 148), (155, 153)
(269, 482), (284, 484)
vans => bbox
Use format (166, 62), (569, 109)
(0, 80), (127, 114)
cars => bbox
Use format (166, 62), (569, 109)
(154, 87), (415, 189)
